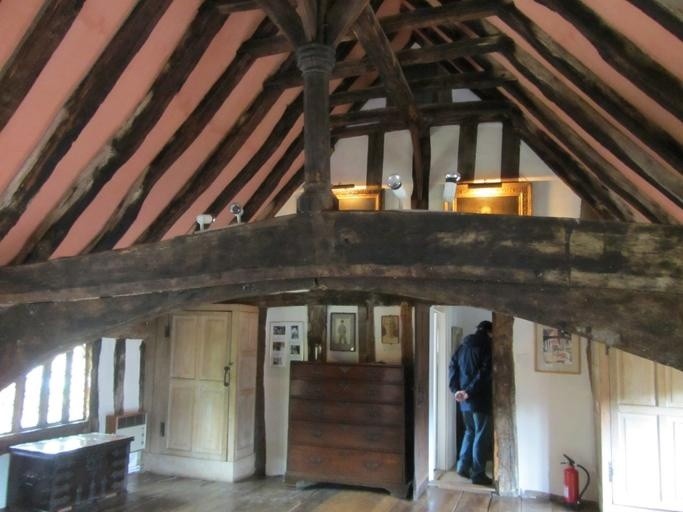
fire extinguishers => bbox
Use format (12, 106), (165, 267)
(560, 454), (590, 508)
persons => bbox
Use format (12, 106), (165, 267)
(382, 315), (399, 344)
(337, 320), (347, 345)
(448, 321), (493, 485)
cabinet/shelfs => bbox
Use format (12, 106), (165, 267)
(4, 431), (134, 512)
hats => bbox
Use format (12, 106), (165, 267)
(475, 320), (492, 329)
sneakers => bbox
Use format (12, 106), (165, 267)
(456, 468), (470, 479)
(471, 475), (492, 484)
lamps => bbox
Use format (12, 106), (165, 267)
(386, 171), (460, 212)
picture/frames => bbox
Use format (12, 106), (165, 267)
(328, 187), (386, 213)
(266, 312), (399, 370)
(442, 179), (533, 217)
(533, 321), (580, 374)
